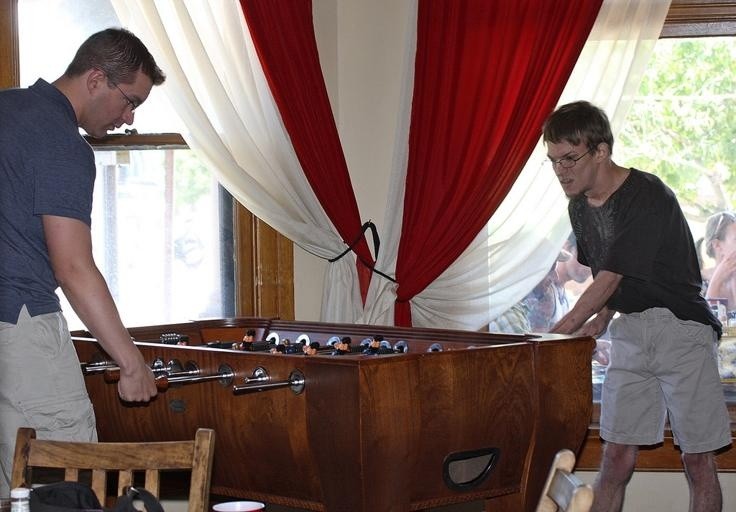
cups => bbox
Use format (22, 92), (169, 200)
(212, 501), (266, 512)
(707, 296), (736, 336)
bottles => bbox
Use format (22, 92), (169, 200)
(8, 487), (31, 512)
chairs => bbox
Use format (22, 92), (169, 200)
(4, 421), (220, 512)
(534, 444), (598, 511)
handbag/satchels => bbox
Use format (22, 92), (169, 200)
(29, 481), (163, 511)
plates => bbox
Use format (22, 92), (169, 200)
(591, 360), (607, 369)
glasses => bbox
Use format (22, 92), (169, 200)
(541, 144), (597, 170)
(712, 209), (736, 240)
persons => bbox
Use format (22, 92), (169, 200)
(537, 99), (729, 512)
(686, 211), (735, 317)
(0, 26), (165, 500)
(547, 227), (592, 335)
(478, 238), (613, 367)
